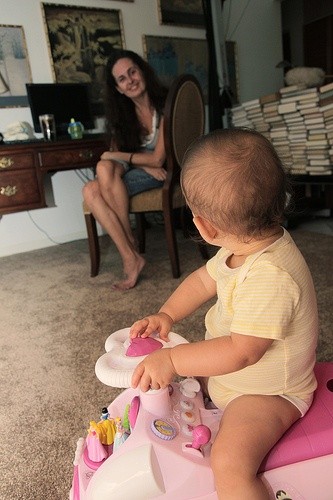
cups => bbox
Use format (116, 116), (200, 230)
(38, 114), (58, 143)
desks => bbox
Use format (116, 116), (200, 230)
(0, 132), (110, 215)
(284, 175), (333, 222)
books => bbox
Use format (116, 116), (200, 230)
(221, 80), (333, 177)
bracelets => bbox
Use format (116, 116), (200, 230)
(129, 152), (135, 164)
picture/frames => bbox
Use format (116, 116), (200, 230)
(143, 34), (240, 106)
(157, 0), (225, 30)
(40, 2), (127, 104)
(0, 24), (33, 109)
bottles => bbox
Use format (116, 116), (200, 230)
(67, 117), (85, 140)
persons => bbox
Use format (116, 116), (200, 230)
(83, 49), (169, 292)
(129, 126), (318, 499)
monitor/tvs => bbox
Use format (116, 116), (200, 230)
(26, 83), (96, 137)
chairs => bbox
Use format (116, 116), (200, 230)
(81, 73), (208, 279)
